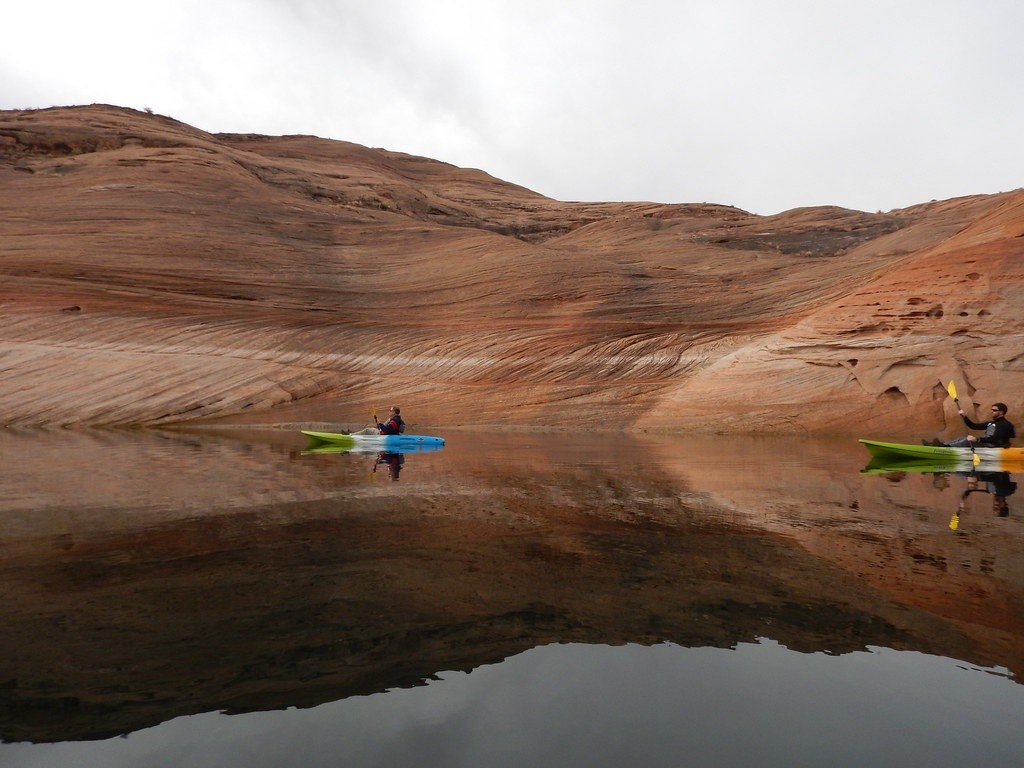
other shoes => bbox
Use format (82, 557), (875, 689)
(341, 429), (354, 435)
(922, 438), (948, 447)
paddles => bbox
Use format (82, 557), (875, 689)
(370, 454), (379, 483)
(948, 380), (981, 466)
(370, 405), (380, 430)
(948, 490), (971, 531)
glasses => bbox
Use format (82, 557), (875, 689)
(991, 409), (1000, 412)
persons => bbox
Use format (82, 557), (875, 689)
(921, 402), (1014, 450)
(341, 405), (401, 437)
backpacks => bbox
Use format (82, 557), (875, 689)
(399, 420), (405, 434)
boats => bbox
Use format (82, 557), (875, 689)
(301, 443), (442, 457)
(300, 429), (446, 446)
(859, 457), (1023, 477)
(858, 439), (1024, 461)
